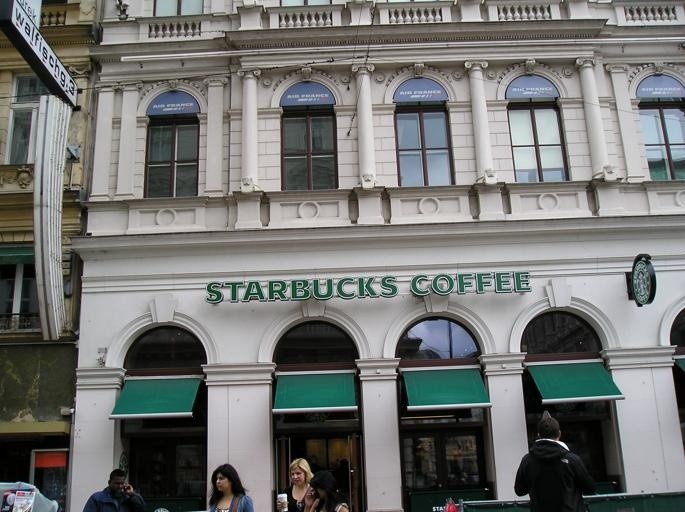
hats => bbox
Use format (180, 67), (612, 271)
(310, 470), (337, 492)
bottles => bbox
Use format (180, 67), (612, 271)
(443, 497), (458, 512)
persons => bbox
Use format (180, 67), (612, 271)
(304, 472), (348, 511)
(209, 464), (254, 512)
(83, 469), (147, 512)
(276, 458), (317, 511)
(514, 419), (595, 512)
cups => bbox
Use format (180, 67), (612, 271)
(277, 493), (288, 512)
